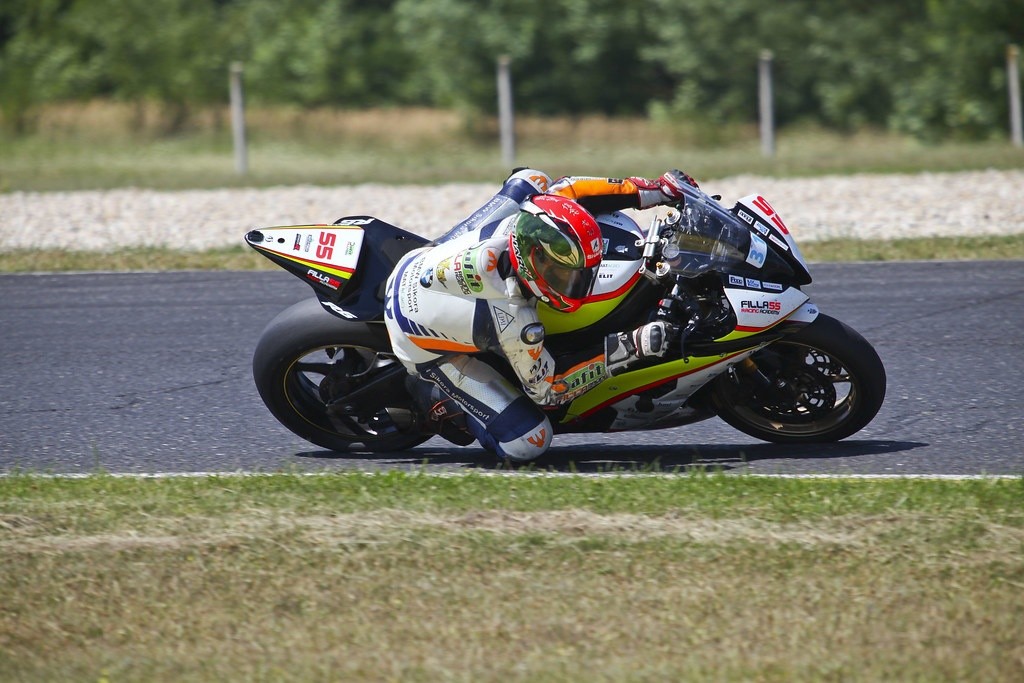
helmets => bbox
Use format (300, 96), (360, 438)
(509, 194), (603, 313)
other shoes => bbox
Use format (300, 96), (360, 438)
(405, 375), (475, 445)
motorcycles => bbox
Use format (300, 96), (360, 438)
(239, 170), (890, 457)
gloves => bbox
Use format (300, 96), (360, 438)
(604, 322), (674, 377)
(626, 169), (700, 210)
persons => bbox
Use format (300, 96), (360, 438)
(383, 166), (699, 463)
